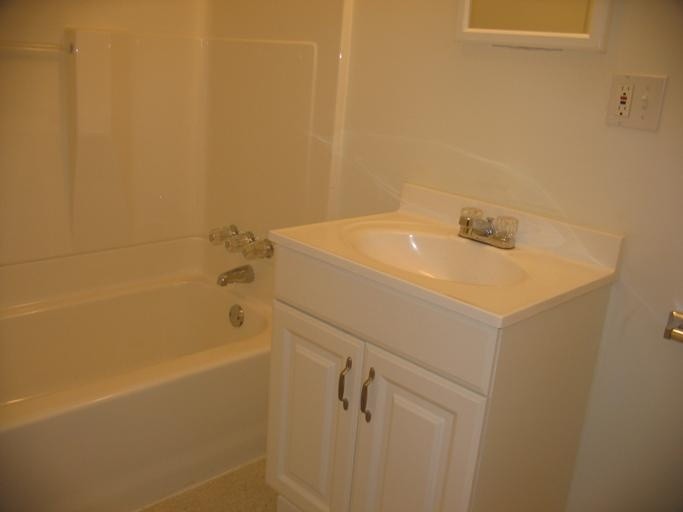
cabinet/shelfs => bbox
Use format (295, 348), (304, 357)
(258, 282), (613, 512)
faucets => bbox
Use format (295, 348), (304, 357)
(458, 207), (519, 249)
(218, 265), (255, 287)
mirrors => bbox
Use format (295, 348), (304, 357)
(456, 1), (620, 55)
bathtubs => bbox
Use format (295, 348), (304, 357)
(0, 278), (271, 512)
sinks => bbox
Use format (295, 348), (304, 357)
(350, 221), (523, 285)
